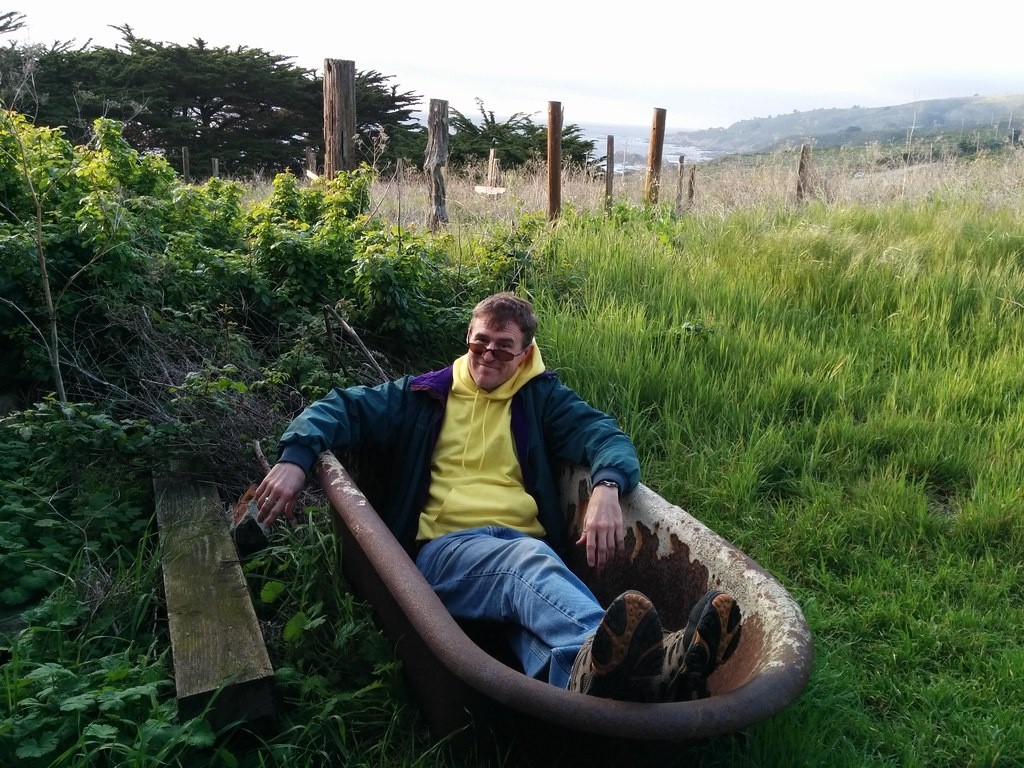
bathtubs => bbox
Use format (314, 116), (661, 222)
(316, 451), (809, 768)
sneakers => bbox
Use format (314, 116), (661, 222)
(648, 589), (742, 702)
(570, 589), (663, 702)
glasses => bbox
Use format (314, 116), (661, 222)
(467, 334), (529, 361)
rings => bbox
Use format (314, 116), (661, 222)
(265, 498), (274, 505)
(583, 530), (586, 533)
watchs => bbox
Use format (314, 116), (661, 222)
(598, 479), (618, 487)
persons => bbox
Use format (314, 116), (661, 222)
(255, 293), (741, 700)
